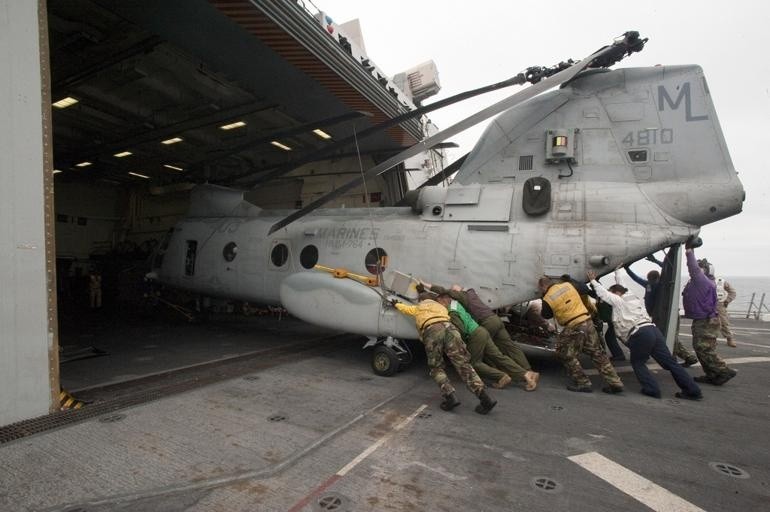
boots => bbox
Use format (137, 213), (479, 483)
(475, 387), (497, 414)
(440, 392), (461, 411)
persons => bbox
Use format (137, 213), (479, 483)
(717, 281), (736, 347)
(502, 300), (555, 333)
(386, 291), (498, 414)
(560, 274), (604, 338)
(585, 263), (703, 400)
(536, 277), (624, 393)
(681, 238), (739, 387)
(591, 284), (627, 361)
(620, 256), (698, 367)
(415, 282), (540, 391)
(88, 267), (104, 309)
(417, 277), (534, 373)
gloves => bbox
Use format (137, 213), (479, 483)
(390, 298), (402, 309)
(416, 283), (426, 293)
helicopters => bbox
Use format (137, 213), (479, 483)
(137, 30), (745, 378)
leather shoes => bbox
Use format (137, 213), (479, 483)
(693, 375), (711, 383)
(602, 386), (625, 393)
(641, 388), (661, 398)
(567, 384), (592, 392)
(524, 371), (540, 391)
(711, 366), (737, 385)
(675, 391), (704, 400)
(492, 374), (512, 388)
(682, 355), (699, 368)
(727, 338), (736, 347)
